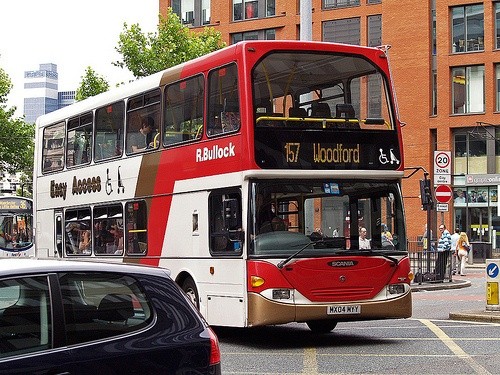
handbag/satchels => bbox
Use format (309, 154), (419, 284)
(414, 271), (438, 282)
(462, 241), (471, 251)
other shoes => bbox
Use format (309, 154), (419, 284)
(461, 274), (466, 276)
(458, 271), (460, 274)
(453, 272), (455, 275)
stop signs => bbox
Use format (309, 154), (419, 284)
(436, 185), (454, 204)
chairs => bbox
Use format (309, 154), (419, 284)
(0, 281), (135, 358)
(154, 98), (361, 151)
(73, 130), (138, 166)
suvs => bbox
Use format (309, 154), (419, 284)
(0, 259), (222, 375)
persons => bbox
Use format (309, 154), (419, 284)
(70, 220), (148, 255)
(1, 226), (33, 243)
(333, 226), (340, 237)
(93, 134), (126, 161)
(306, 226), (325, 236)
(454, 188), (499, 203)
(437, 223), (452, 275)
(381, 223), (395, 246)
(129, 117), (159, 152)
(454, 232), (470, 277)
(358, 226), (364, 236)
(450, 228), (462, 275)
(420, 222), (437, 257)
(358, 227), (371, 250)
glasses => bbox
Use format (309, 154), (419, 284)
(148, 125), (154, 129)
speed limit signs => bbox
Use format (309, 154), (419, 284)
(435, 150), (452, 173)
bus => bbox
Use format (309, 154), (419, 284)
(33, 39), (435, 336)
(0, 196), (35, 259)
